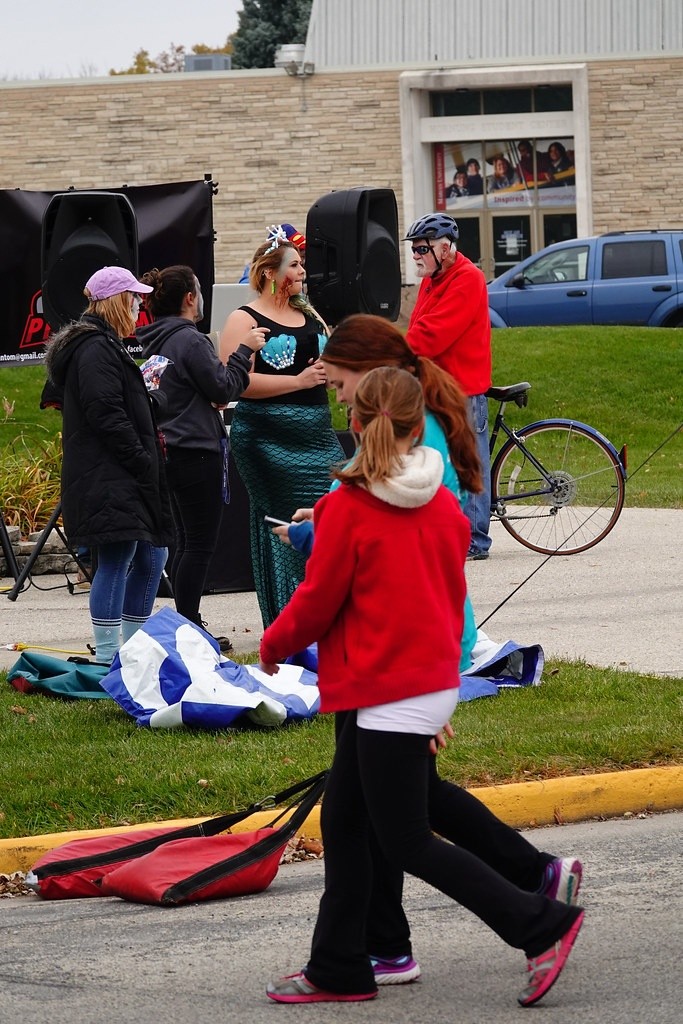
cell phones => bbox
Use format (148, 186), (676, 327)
(264, 516), (290, 526)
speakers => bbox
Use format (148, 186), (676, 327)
(39, 193), (139, 333)
(306, 186), (402, 324)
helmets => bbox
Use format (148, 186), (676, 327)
(401, 212), (459, 241)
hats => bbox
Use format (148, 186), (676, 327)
(85, 266), (154, 302)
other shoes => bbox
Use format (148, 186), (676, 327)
(465, 550), (489, 560)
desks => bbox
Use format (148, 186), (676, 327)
(210, 282), (306, 353)
(157, 430), (366, 599)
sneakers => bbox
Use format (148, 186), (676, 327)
(368, 954), (422, 985)
(265, 972), (377, 1003)
(538, 857), (582, 905)
(516, 910), (586, 1006)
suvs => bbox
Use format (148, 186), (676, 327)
(485, 229), (683, 327)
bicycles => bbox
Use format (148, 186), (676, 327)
(484, 381), (628, 556)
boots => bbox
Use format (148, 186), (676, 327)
(183, 612), (232, 650)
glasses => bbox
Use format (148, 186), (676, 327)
(412, 243), (441, 254)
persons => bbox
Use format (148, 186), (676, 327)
(405, 214), (492, 562)
(445, 141), (576, 199)
(221, 224), (346, 636)
(239, 224), (306, 302)
(260, 314), (587, 1007)
(40, 265), (179, 665)
(134, 265), (271, 651)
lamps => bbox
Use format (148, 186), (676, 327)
(285, 60), (315, 78)
